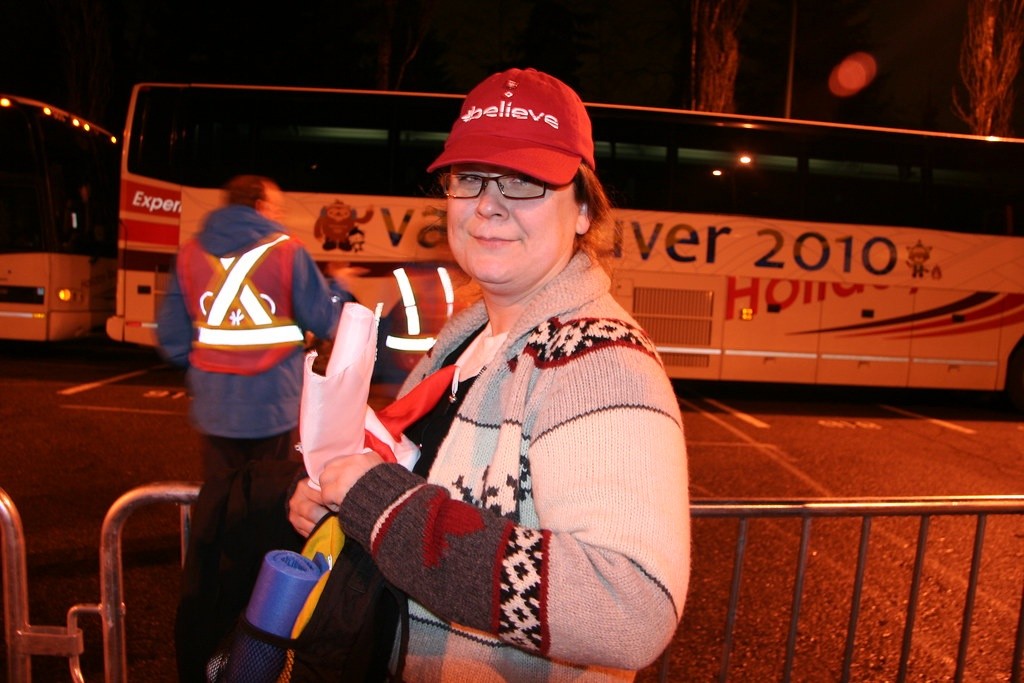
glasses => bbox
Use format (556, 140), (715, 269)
(441, 173), (547, 200)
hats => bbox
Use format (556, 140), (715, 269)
(424, 67), (596, 188)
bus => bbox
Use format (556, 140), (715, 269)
(105, 79), (1023, 407)
(0, 95), (122, 345)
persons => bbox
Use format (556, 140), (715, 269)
(289, 64), (694, 683)
(154, 174), (363, 623)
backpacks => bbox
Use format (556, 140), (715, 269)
(193, 511), (411, 682)
